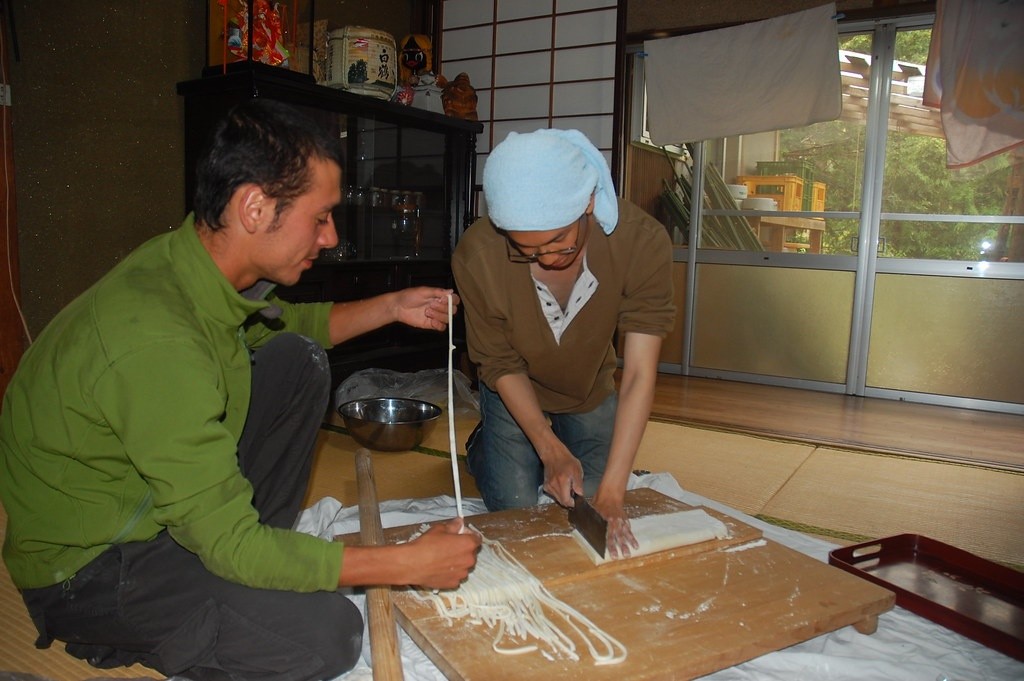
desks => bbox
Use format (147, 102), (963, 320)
(741, 208), (825, 254)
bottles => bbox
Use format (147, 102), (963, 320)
(344, 185), (424, 261)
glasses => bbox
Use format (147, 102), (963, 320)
(506, 218), (580, 264)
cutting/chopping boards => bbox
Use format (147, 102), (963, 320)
(333, 487), (896, 681)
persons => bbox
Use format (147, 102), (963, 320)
(0, 101), (483, 681)
(450, 128), (674, 557)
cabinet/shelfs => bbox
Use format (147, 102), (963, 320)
(174, 69), (485, 408)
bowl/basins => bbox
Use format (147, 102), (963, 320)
(337, 397), (443, 452)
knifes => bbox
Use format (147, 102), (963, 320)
(568, 489), (608, 559)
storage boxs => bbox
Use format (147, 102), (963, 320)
(756, 191), (812, 211)
(755, 160), (814, 199)
(735, 175), (805, 212)
(811, 181), (827, 222)
